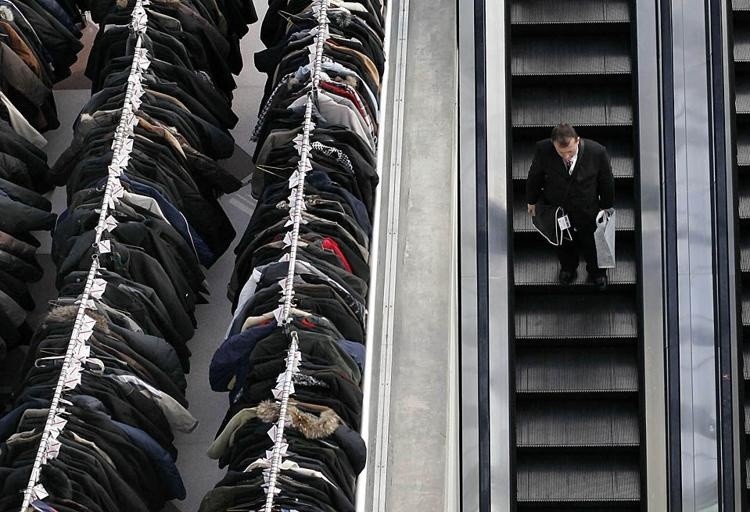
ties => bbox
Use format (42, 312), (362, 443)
(565, 160), (573, 172)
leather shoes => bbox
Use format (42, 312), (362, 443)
(559, 269), (571, 287)
(594, 271), (606, 291)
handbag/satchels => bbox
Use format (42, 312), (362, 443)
(530, 178), (576, 247)
(595, 207), (616, 269)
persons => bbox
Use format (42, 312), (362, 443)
(526, 123), (616, 291)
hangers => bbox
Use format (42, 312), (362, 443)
(259, 1), (331, 511)
(19, 1), (148, 512)
